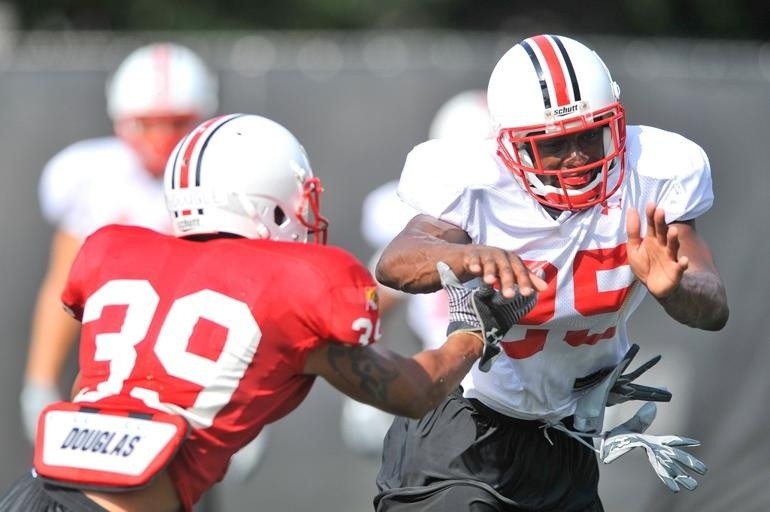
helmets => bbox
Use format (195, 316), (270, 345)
(163, 113), (329, 241)
(490, 35), (626, 212)
(107, 43), (219, 173)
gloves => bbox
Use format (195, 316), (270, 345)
(572, 344), (672, 433)
(599, 403), (706, 492)
(434, 262), (545, 351)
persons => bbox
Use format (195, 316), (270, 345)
(1, 110), (551, 510)
(352, 80), (684, 465)
(372, 31), (730, 510)
(16, 39), (269, 489)
